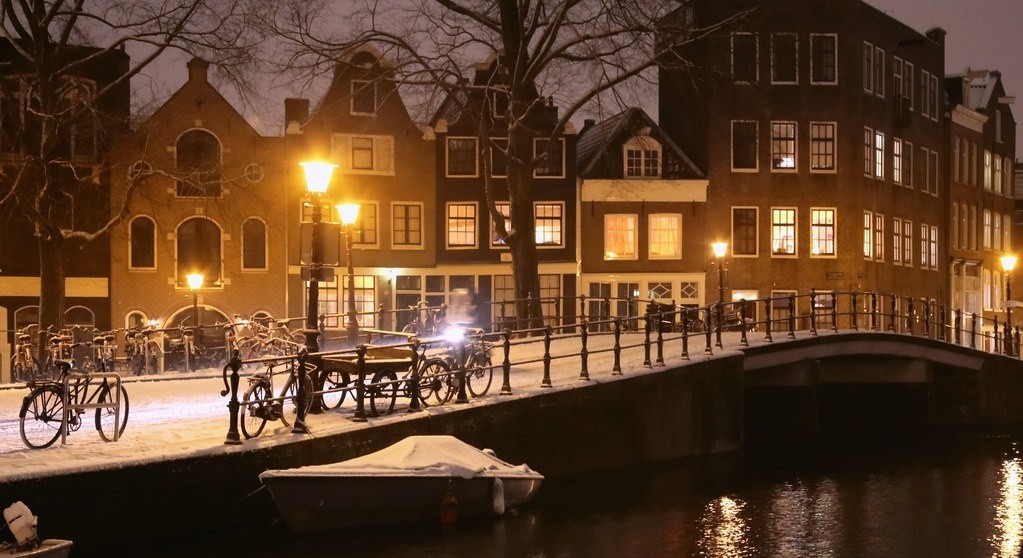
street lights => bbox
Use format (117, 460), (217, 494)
(709, 237), (726, 322)
(295, 150), (341, 410)
(185, 269), (204, 361)
(1000, 254), (1017, 327)
(331, 200), (362, 352)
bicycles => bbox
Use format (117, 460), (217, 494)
(399, 301), (451, 339)
(672, 314), (758, 334)
(239, 343), (317, 443)
(39, 313), (306, 380)
(8, 328), (43, 384)
(320, 321), (496, 418)
(19, 335), (130, 449)
